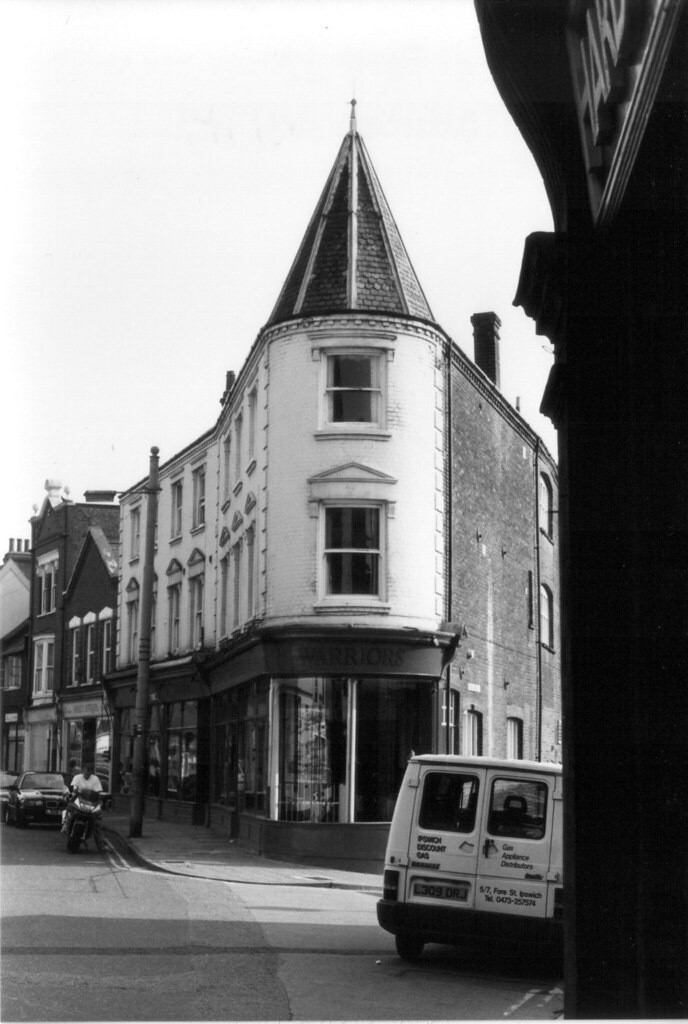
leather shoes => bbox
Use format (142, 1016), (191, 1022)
(100, 848), (112, 854)
(62, 825), (68, 832)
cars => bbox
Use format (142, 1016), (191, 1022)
(0, 771), (21, 804)
(4, 771), (69, 826)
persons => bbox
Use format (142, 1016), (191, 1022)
(60, 765), (108, 850)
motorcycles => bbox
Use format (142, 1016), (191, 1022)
(63, 784), (111, 855)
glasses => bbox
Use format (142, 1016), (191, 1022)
(85, 773), (91, 776)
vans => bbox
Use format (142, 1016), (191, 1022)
(377, 753), (561, 977)
